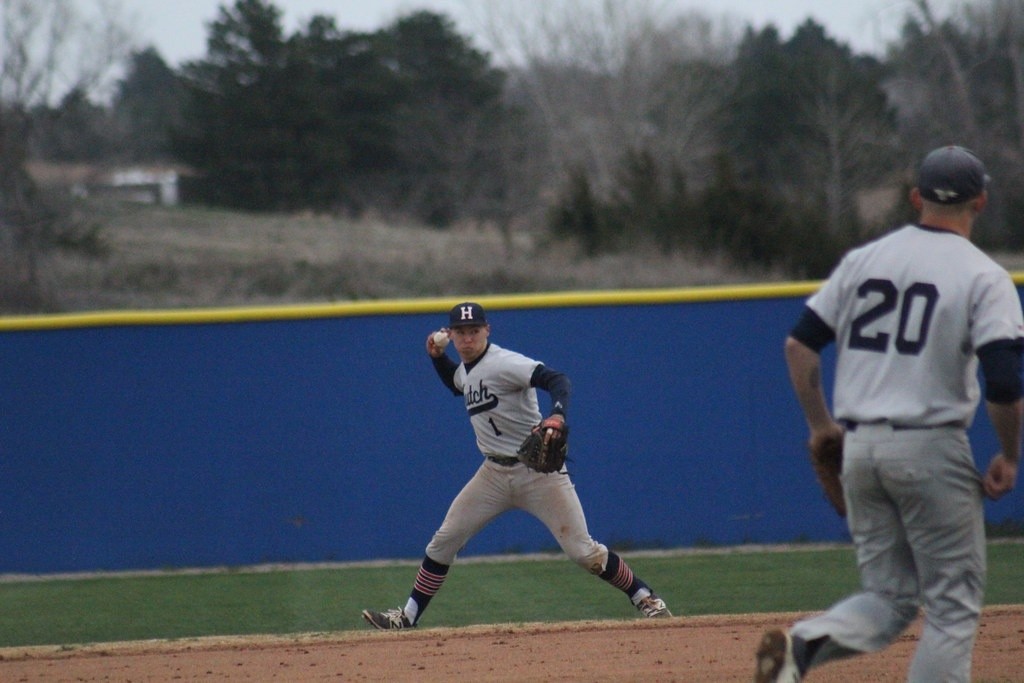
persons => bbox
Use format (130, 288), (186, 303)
(753, 147), (1024, 683)
(362, 302), (673, 629)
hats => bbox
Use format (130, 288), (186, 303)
(448, 302), (487, 329)
(917, 145), (991, 205)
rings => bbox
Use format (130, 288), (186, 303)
(546, 428), (553, 434)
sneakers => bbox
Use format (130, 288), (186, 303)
(629, 581), (673, 619)
(754, 628), (809, 683)
(361, 606), (419, 632)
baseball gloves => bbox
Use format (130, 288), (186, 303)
(517, 418), (569, 475)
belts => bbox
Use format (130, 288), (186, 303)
(488, 456), (519, 466)
(836, 418), (966, 433)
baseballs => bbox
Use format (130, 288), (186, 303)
(433, 330), (449, 349)
